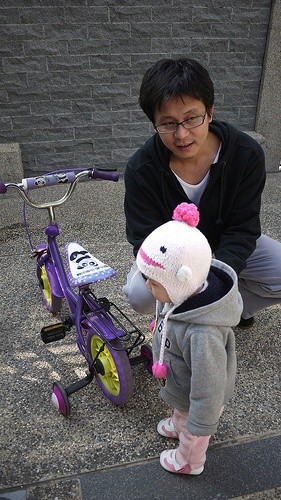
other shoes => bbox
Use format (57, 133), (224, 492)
(237, 315), (254, 329)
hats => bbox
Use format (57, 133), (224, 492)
(135, 202), (212, 379)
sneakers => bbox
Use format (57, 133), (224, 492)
(157, 418), (179, 438)
(160, 449), (204, 475)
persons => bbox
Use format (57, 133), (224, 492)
(124, 58), (281, 330)
(135, 201), (243, 475)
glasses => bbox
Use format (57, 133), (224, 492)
(154, 106), (207, 133)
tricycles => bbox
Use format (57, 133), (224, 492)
(0, 166), (154, 419)
